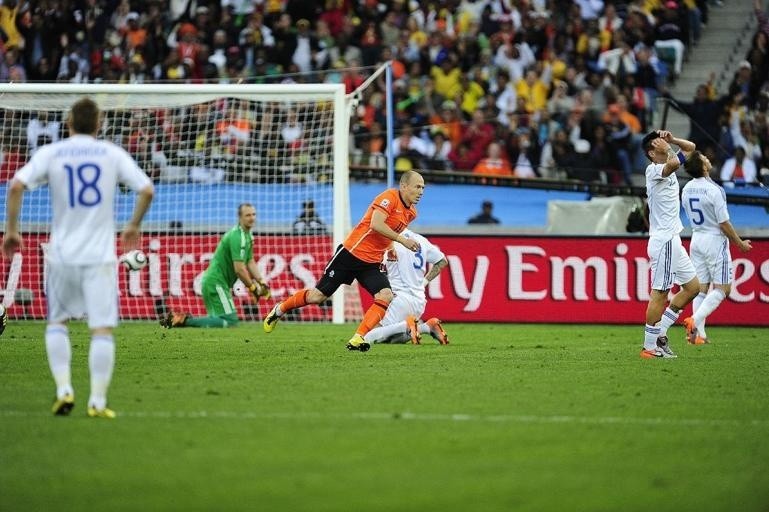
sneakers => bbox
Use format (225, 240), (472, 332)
(406, 316), (420, 345)
(684, 316), (710, 343)
(264, 300), (288, 334)
(426, 318), (449, 345)
(51, 393), (75, 417)
(640, 335), (679, 360)
(160, 312), (191, 328)
(346, 337), (371, 352)
(87, 407), (116, 418)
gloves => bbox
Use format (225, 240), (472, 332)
(246, 284), (260, 304)
(257, 278), (271, 300)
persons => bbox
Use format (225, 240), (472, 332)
(263, 169), (425, 352)
(363, 227), (450, 346)
(681, 150), (754, 345)
(168, 203), (271, 329)
(638, 130), (701, 359)
(3, 99), (155, 418)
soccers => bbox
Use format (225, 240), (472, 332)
(124, 251), (147, 270)
